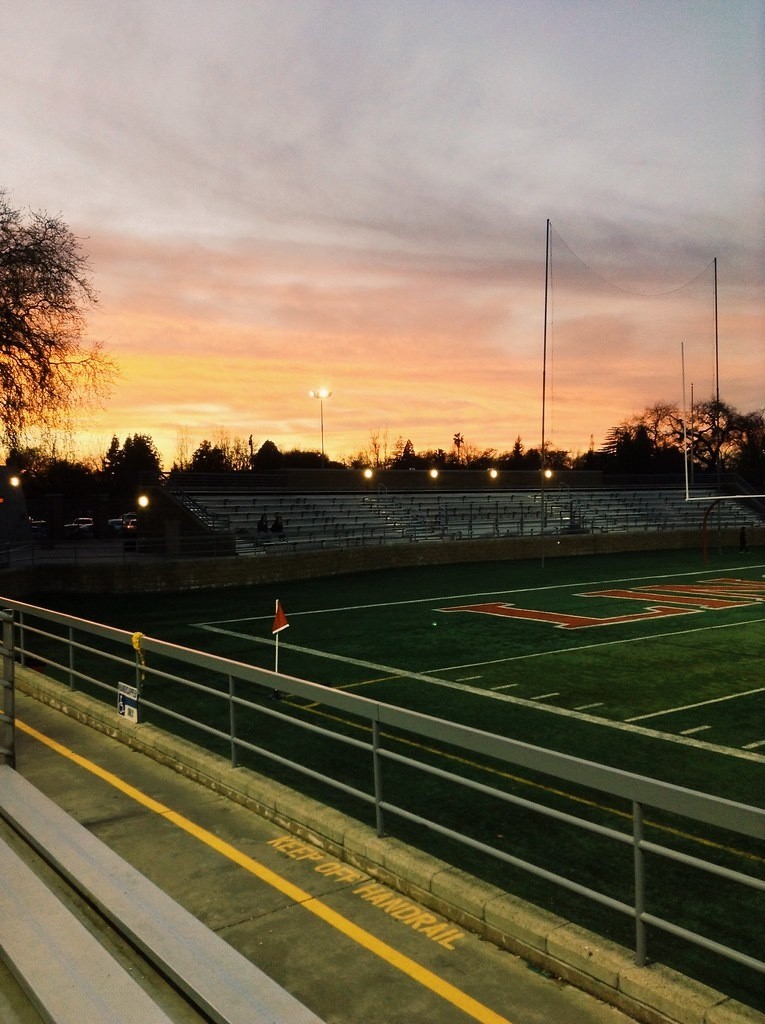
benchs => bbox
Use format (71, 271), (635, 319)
(176, 489), (765, 553)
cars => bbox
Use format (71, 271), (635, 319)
(27, 516), (139, 538)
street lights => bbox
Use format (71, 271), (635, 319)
(310, 389), (333, 469)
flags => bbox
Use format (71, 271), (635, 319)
(272, 602), (290, 635)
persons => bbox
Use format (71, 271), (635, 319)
(272, 517), (283, 533)
(257, 514), (268, 532)
(738, 527), (751, 553)
